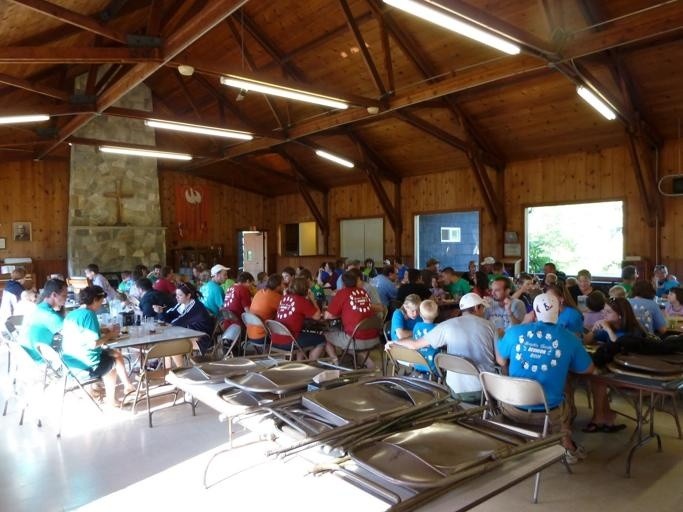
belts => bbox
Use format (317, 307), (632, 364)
(512, 405), (560, 415)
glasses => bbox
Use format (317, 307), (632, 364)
(174, 282), (186, 287)
(96, 292), (107, 296)
(608, 296), (620, 313)
(655, 265), (665, 269)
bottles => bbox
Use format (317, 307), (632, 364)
(135, 314), (141, 326)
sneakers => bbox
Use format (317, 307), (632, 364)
(564, 445), (587, 465)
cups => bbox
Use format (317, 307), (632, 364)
(147, 316), (154, 333)
(102, 314), (108, 324)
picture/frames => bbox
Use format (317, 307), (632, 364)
(11, 220), (33, 242)
(0, 237), (7, 250)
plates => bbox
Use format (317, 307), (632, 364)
(109, 334), (129, 340)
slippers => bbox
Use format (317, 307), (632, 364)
(583, 421), (606, 434)
(603, 423), (629, 432)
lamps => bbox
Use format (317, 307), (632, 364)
(382, 0), (521, 57)
(0, 114), (51, 126)
(143, 117), (254, 141)
(219, 72), (350, 110)
(577, 82), (617, 122)
(97, 144), (192, 162)
(315, 148), (355, 169)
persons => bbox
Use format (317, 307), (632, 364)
(87, 258), (441, 367)
(0, 268), (35, 331)
(19, 278), (68, 363)
(15, 225), (29, 240)
(385, 257), (682, 461)
(60, 285), (136, 407)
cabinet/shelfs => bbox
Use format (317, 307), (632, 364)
(173, 249), (215, 269)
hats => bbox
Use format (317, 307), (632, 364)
(533, 294), (561, 325)
(458, 292), (490, 311)
(480, 256), (497, 265)
(565, 277), (579, 288)
(210, 264), (231, 277)
